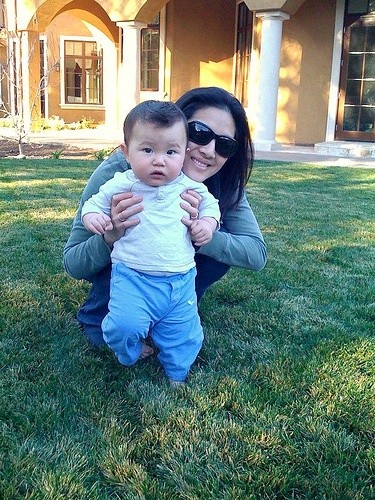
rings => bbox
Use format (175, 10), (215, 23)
(191, 211), (200, 220)
(116, 213), (126, 222)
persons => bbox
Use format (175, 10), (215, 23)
(80, 99), (222, 392)
(62, 85), (269, 348)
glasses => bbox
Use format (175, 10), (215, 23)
(187, 119), (238, 158)
